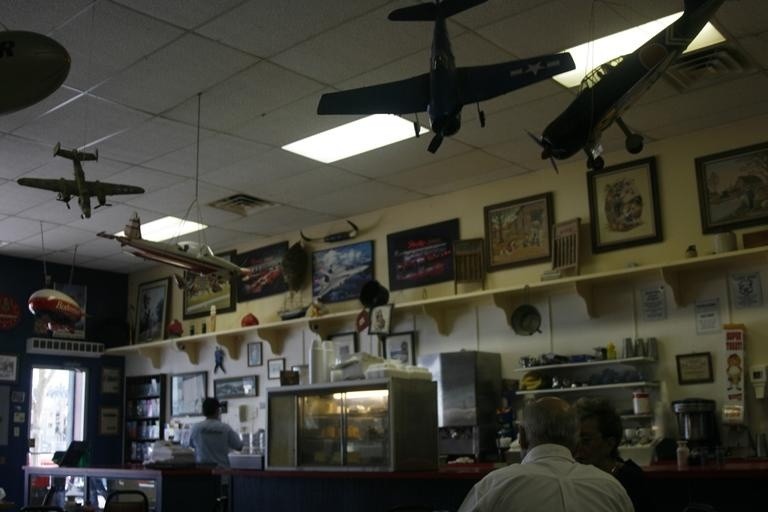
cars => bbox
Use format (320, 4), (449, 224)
(27, 427), (63, 488)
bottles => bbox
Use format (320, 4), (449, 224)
(188, 305), (217, 335)
(674, 438), (691, 471)
(590, 336), (658, 361)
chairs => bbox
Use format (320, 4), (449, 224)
(19, 506), (64, 512)
(103, 490), (149, 512)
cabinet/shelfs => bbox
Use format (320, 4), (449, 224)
(263, 378), (438, 473)
(124, 373), (165, 466)
(23, 469), (219, 512)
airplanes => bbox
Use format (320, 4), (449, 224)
(16, 142), (144, 219)
(525, 1), (723, 176)
(317, 0), (580, 158)
(314, 264), (372, 300)
(96, 209), (253, 284)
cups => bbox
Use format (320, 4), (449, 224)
(321, 340), (337, 382)
(309, 338), (324, 383)
(622, 426), (652, 445)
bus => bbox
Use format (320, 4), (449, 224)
(394, 243), (451, 271)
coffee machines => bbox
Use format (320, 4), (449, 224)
(671, 396), (718, 467)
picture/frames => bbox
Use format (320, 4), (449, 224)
(585, 154), (664, 256)
(0, 351), (20, 385)
(182, 249), (236, 320)
(97, 366), (122, 439)
(693, 142), (767, 236)
(483, 191), (555, 273)
(236, 240), (290, 304)
(131, 276), (173, 344)
(311, 239), (376, 303)
(386, 217), (461, 292)
(133, 303), (768, 417)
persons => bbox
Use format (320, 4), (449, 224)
(190, 398), (243, 511)
(457, 396), (635, 512)
(140, 294), (158, 333)
(569, 396), (664, 512)
